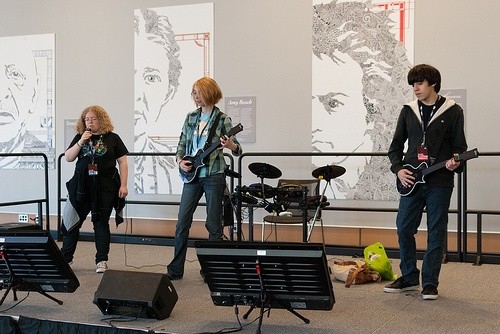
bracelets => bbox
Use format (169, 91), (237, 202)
(77, 142), (82, 147)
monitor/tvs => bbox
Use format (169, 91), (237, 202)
(0, 230), (80, 293)
(194, 240), (336, 311)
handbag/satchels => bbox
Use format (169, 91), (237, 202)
(328, 260), (382, 289)
(364, 241), (398, 281)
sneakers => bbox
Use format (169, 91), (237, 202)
(96, 261), (108, 273)
(421, 285), (439, 300)
(384, 276), (419, 293)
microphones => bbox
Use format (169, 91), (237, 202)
(85, 128), (91, 143)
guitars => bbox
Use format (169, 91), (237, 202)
(396, 147), (480, 197)
(178, 122), (244, 184)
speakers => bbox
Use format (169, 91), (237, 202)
(93, 270), (179, 321)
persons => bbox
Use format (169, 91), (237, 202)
(165, 77), (242, 283)
(384, 64), (468, 299)
(59, 106), (129, 273)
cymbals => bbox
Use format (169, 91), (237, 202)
(248, 184), (276, 198)
(248, 162), (282, 179)
(312, 165), (346, 180)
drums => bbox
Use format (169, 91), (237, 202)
(279, 183), (306, 200)
(305, 194), (328, 203)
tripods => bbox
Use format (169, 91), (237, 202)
(243, 259), (310, 334)
(0, 246), (63, 307)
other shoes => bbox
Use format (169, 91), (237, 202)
(165, 273), (183, 280)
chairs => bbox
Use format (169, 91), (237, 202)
(262, 179), (326, 258)
